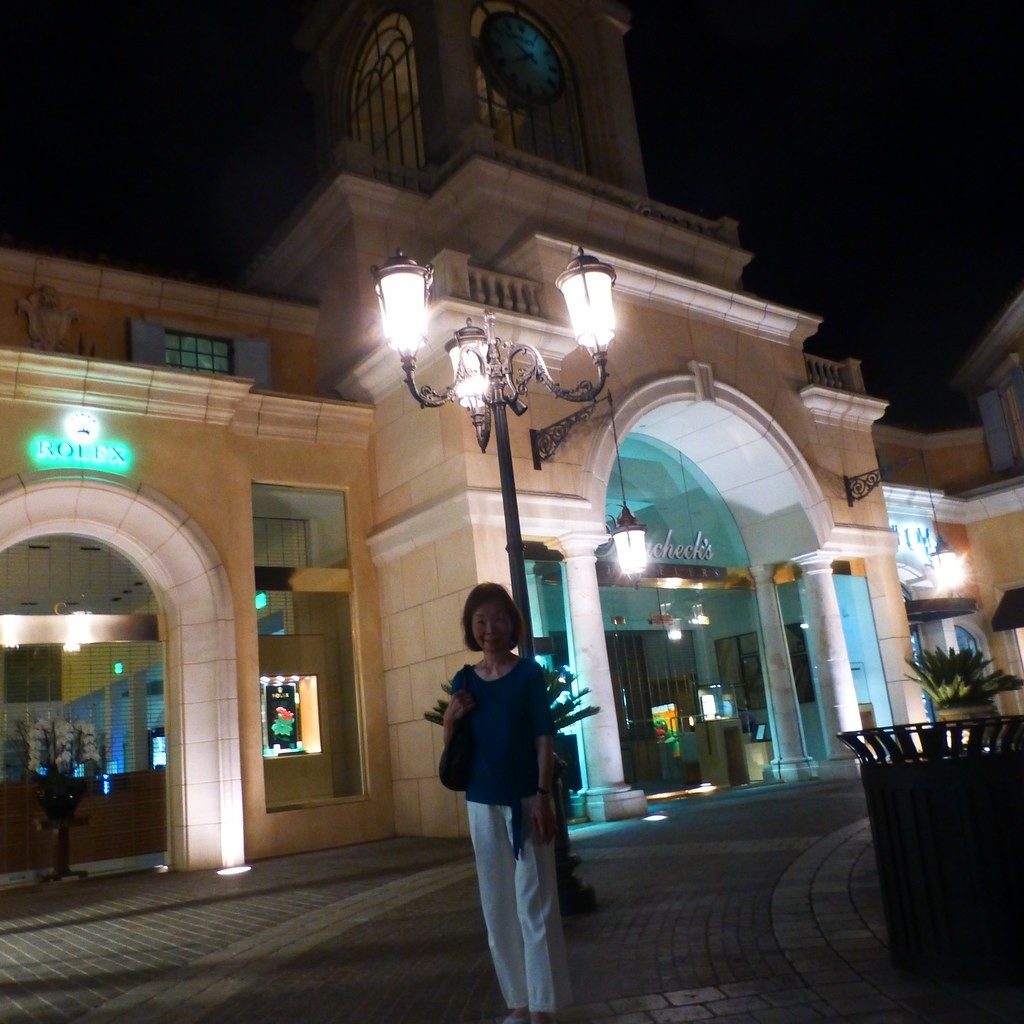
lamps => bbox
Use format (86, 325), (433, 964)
(843, 450), (966, 593)
(532, 389), (648, 576)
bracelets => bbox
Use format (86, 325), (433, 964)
(536, 787), (553, 797)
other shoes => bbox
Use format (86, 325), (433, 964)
(503, 1015), (528, 1024)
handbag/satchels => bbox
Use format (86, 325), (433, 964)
(439, 665), (475, 792)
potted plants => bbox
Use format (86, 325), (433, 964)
(904, 642), (1024, 721)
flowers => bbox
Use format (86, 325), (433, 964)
(4, 707), (119, 778)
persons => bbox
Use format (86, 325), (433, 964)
(438, 583), (579, 1024)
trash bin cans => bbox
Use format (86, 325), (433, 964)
(837, 716), (1024, 980)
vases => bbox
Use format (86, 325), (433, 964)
(35, 774), (87, 820)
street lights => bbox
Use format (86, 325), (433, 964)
(373, 239), (618, 917)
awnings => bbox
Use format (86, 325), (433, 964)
(990, 587), (1024, 633)
